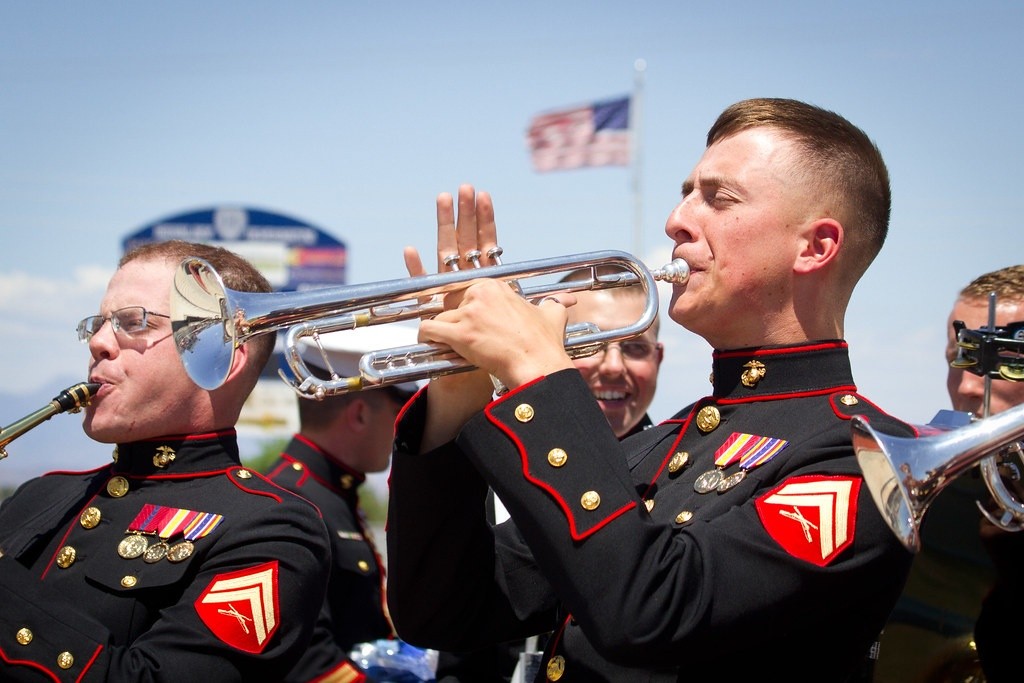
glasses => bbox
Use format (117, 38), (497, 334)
(76, 306), (170, 343)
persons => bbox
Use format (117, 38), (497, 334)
(877, 262), (1024, 683)
(433, 262), (666, 683)
(0, 239), (332, 683)
(387, 97), (919, 683)
(261, 319), (427, 683)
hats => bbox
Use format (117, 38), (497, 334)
(298, 325), (425, 401)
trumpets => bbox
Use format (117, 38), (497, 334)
(848, 401), (1024, 557)
(167, 246), (693, 403)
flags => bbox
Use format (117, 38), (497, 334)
(526, 94), (632, 173)
